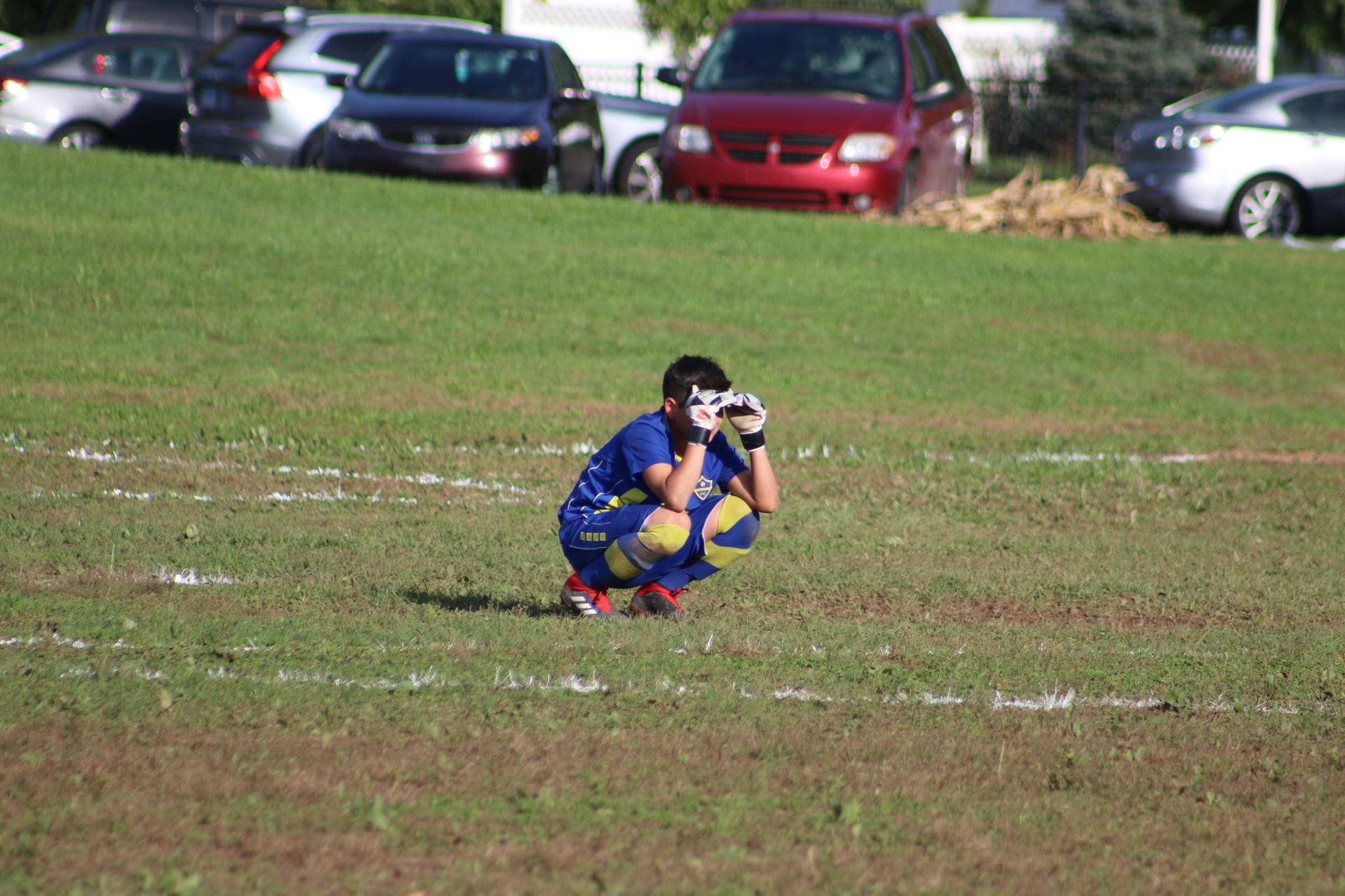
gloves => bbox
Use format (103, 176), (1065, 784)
(685, 384), (737, 446)
(724, 392), (766, 453)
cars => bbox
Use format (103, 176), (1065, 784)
(0, 0), (310, 155)
(323, 30), (606, 195)
(1117, 71), (1345, 243)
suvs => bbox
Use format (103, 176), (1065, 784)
(179, 7), (676, 204)
(655, 2), (977, 219)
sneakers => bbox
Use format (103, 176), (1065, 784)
(629, 581), (685, 617)
(558, 571), (630, 622)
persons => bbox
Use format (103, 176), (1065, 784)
(557, 353), (780, 620)
